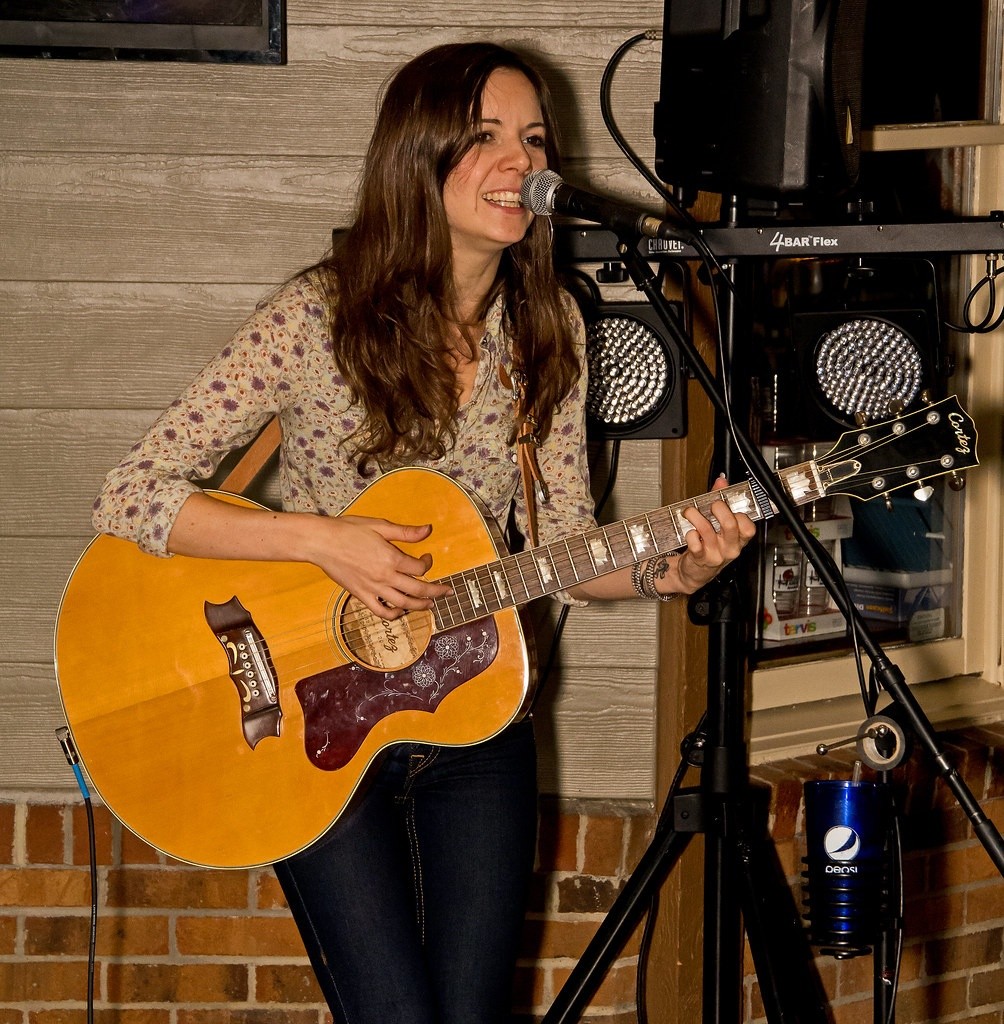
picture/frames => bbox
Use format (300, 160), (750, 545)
(0, 0), (288, 67)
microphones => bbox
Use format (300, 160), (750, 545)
(521, 167), (693, 246)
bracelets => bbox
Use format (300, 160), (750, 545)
(631, 551), (682, 602)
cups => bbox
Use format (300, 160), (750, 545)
(802, 781), (887, 947)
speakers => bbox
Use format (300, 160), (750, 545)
(656, 0), (836, 197)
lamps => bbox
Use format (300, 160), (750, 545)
(794, 308), (936, 442)
(581, 299), (688, 439)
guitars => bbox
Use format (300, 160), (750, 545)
(51, 394), (984, 874)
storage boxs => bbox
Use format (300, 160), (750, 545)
(843, 568), (953, 642)
(755, 492), (855, 649)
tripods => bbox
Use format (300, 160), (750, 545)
(541, 195), (835, 1024)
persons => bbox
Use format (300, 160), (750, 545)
(92, 41), (756, 1024)
